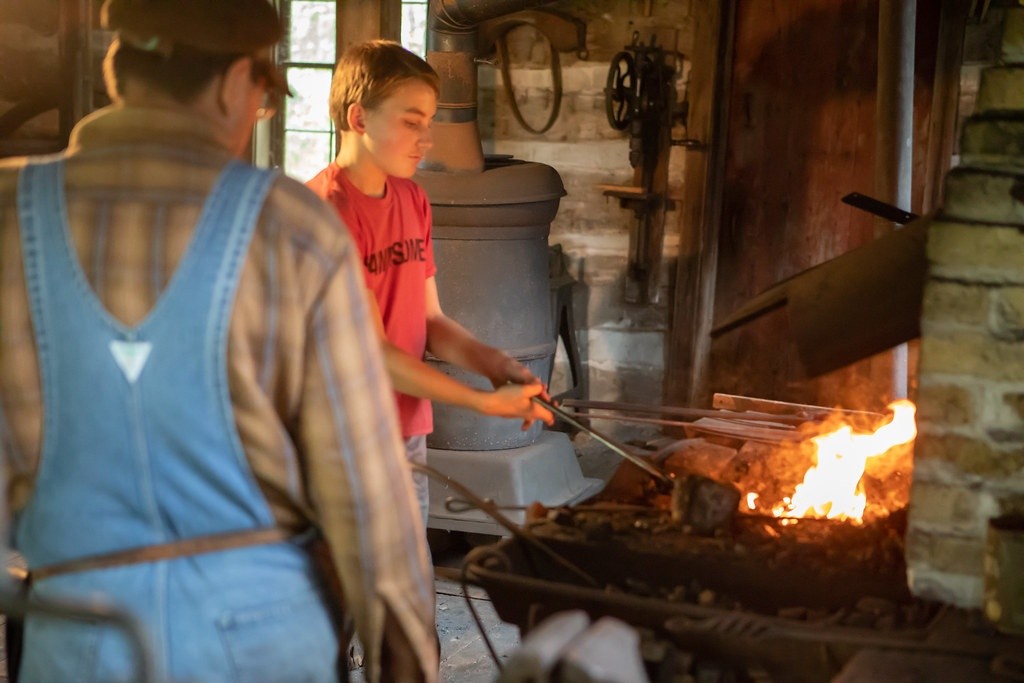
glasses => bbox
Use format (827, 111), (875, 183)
(256, 86), (282, 120)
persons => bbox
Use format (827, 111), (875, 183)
(0, 0), (443, 681)
(306, 39), (556, 568)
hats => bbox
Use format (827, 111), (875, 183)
(100, 0), (294, 98)
